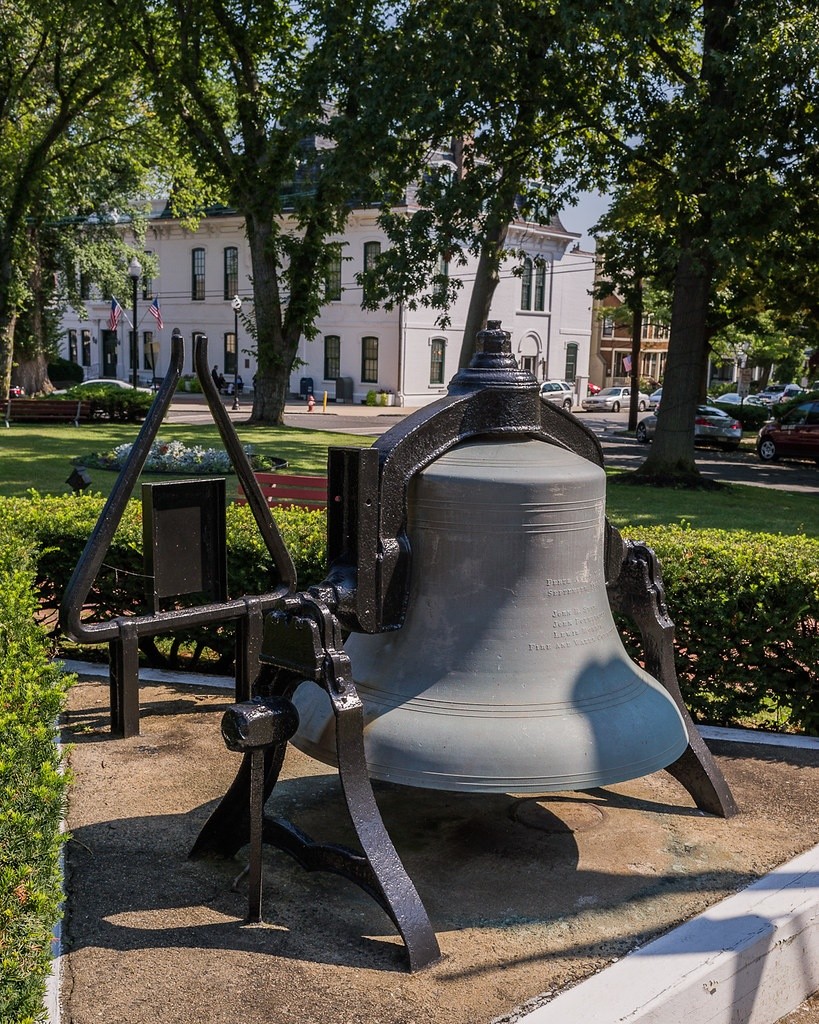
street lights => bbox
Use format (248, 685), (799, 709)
(230, 294), (244, 411)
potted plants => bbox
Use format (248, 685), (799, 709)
(365, 388), (395, 406)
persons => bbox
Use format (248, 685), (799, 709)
(211, 365), (243, 394)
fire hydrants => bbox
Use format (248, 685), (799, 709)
(308, 395), (315, 412)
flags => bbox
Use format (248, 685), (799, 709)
(148, 298), (164, 331)
(107, 300), (122, 331)
(623, 355), (632, 372)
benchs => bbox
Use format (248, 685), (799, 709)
(147, 377), (164, 390)
(220, 382), (244, 396)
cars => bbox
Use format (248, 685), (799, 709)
(635, 404), (744, 451)
(52, 377), (153, 414)
(581, 386), (650, 413)
(650, 388), (664, 409)
(756, 398), (819, 462)
(715, 393), (768, 418)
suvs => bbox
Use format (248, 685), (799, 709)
(538, 379), (574, 414)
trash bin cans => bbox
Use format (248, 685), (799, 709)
(300, 377), (314, 400)
(336, 377), (354, 404)
